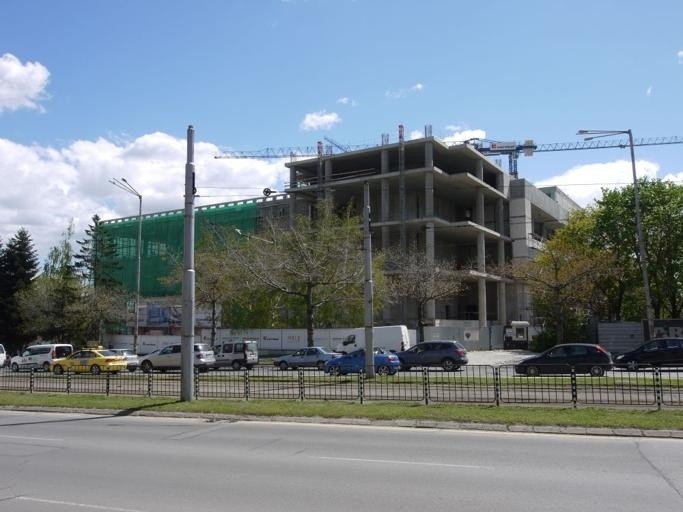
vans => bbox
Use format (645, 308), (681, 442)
(0, 342), (7, 368)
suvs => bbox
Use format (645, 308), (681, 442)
(209, 340), (260, 372)
(8, 343), (73, 373)
(109, 347), (138, 371)
(136, 342), (216, 375)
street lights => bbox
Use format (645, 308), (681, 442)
(574, 127), (656, 339)
(107, 175), (145, 352)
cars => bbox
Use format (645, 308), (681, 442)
(612, 337), (683, 370)
(271, 345), (342, 371)
(49, 345), (128, 375)
(321, 346), (400, 377)
(513, 341), (614, 378)
(394, 338), (470, 372)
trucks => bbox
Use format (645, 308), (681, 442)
(334, 324), (409, 355)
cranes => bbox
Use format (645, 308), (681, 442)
(212, 124), (379, 163)
(453, 127), (682, 181)
